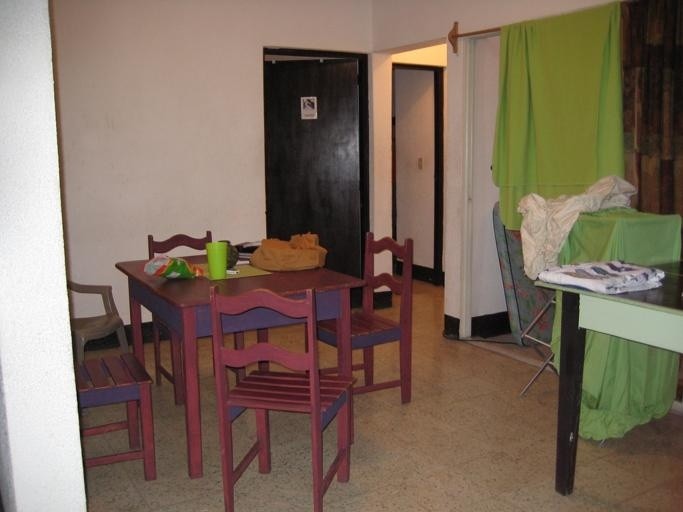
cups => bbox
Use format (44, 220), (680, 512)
(205, 241), (229, 281)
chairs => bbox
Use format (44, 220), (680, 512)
(209, 283), (359, 512)
(68, 278), (157, 483)
(146, 231), (222, 405)
(304, 227), (414, 444)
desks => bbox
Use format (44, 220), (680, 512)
(114, 250), (368, 481)
(536, 261), (683, 498)
